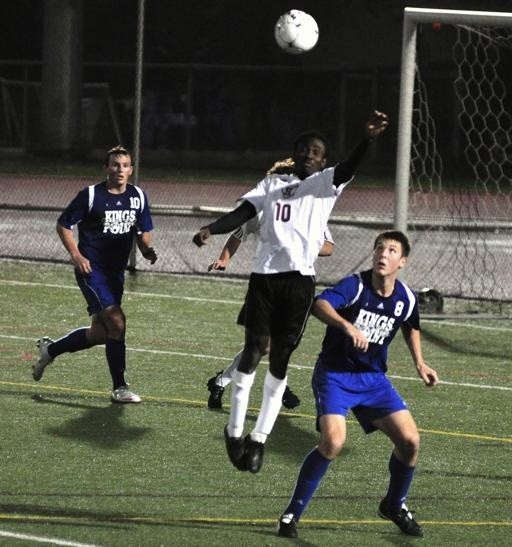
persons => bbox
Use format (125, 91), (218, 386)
(31, 145), (158, 404)
(204, 156), (338, 410)
(277, 228), (440, 540)
(192, 106), (391, 476)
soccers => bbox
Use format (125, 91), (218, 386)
(274, 9), (319, 53)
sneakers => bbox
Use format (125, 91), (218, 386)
(110, 382), (142, 403)
(278, 510), (298, 538)
(32, 336), (54, 381)
(377, 498), (424, 537)
(224, 424), (248, 471)
(282, 385), (300, 409)
(244, 432), (265, 474)
(207, 377), (224, 408)
